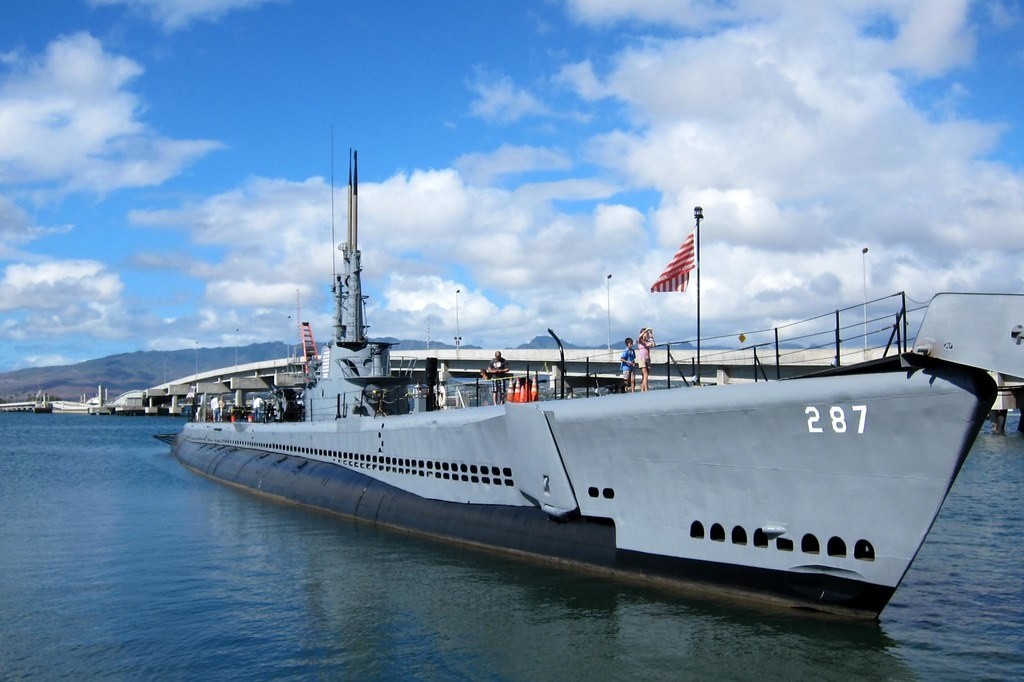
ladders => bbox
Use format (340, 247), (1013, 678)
(300, 322), (319, 375)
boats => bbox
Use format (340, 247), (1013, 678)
(89, 384), (116, 415)
(35, 393), (53, 414)
(152, 124), (1024, 622)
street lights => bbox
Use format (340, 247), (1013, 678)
(455, 288), (460, 348)
(162, 313), (292, 384)
(606, 274), (612, 361)
(861, 247), (870, 360)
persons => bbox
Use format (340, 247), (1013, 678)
(637, 328), (656, 392)
(253, 396), (264, 421)
(488, 351), (510, 406)
(413, 380), (426, 397)
(480, 369), (489, 406)
(619, 337), (636, 393)
(211, 396), (226, 422)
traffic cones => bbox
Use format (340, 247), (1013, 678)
(507, 376), (538, 403)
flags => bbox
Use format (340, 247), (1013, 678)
(650, 231), (696, 293)
